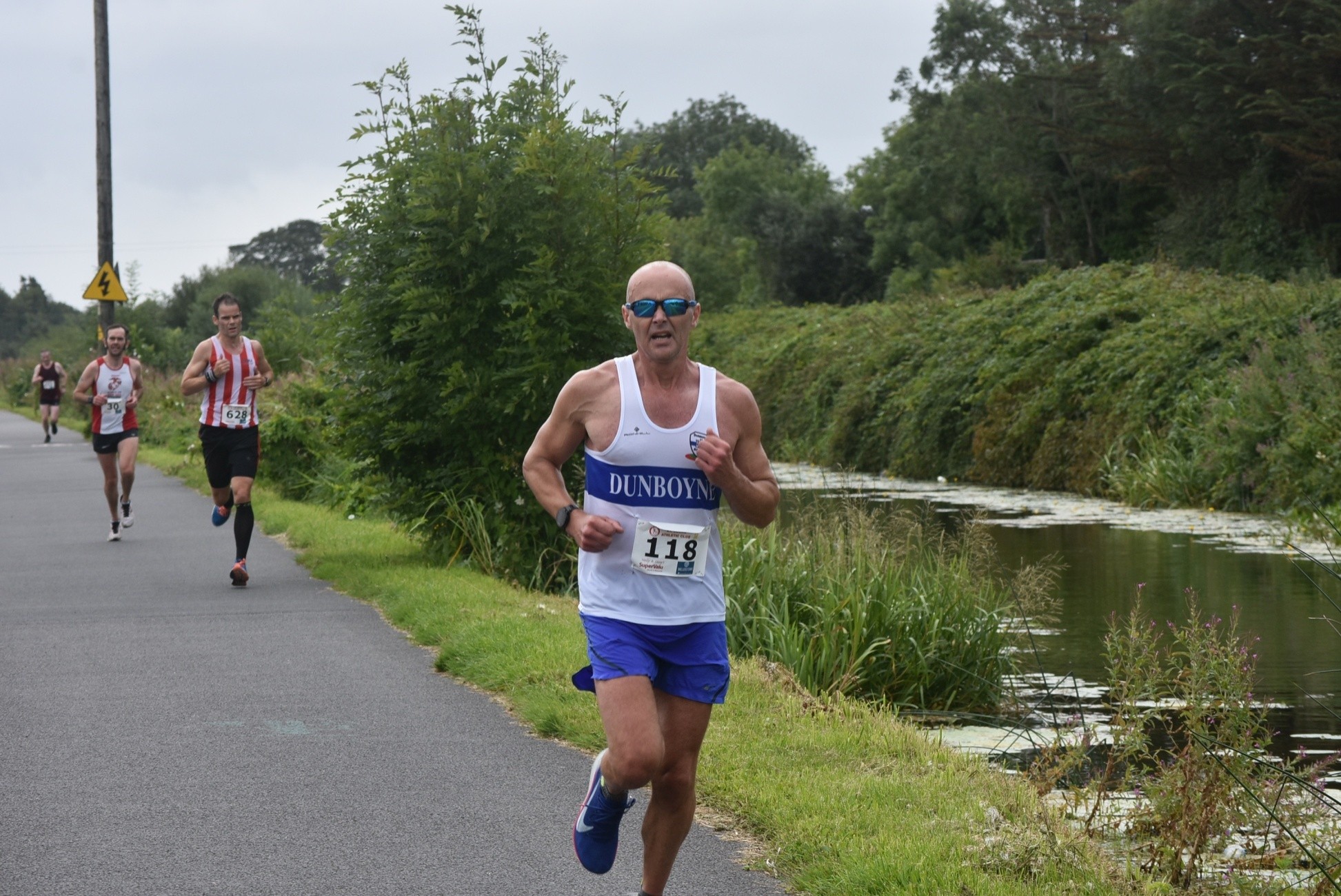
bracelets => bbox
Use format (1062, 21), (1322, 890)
(205, 370), (218, 383)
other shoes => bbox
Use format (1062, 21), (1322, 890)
(44, 435), (50, 443)
(51, 425), (58, 435)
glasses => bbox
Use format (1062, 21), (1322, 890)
(626, 298), (697, 318)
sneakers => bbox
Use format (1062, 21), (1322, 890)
(574, 748), (636, 874)
(120, 495), (134, 528)
(230, 558), (249, 586)
(211, 485), (234, 526)
(107, 518), (121, 541)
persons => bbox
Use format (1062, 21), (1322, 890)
(73, 325), (144, 542)
(32, 348), (68, 444)
(522, 260), (780, 896)
(180, 293), (274, 585)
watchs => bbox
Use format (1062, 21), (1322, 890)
(264, 376), (269, 387)
(555, 504), (579, 532)
(88, 395), (93, 404)
